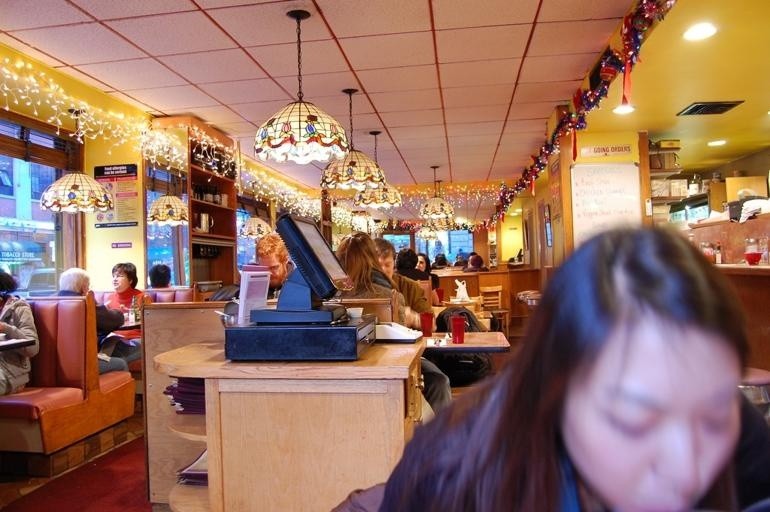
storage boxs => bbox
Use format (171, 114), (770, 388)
(668, 179), (688, 197)
(651, 179), (670, 198)
(655, 139), (682, 151)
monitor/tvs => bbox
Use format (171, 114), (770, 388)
(250, 213), (354, 322)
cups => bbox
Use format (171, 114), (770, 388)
(742, 236), (769, 265)
(348, 308), (365, 317)
(420, 286), (466, 345)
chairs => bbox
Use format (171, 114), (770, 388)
(441, 300), (478, 313)
(449, 295), (484, 312)
(479, 285), (510, 339)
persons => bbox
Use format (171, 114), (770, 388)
(1, 266), (40, 397)
(50, 266), (130, 375)
(338, 230), (490, 419)
(234, 234), (295, 299)
(330, 222), (769, 509)
(97, 262), (155, 364)
(149, 263), (170, 289)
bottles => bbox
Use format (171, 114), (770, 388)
(192, 147), (229, 232)
(688, 169), (742, 193)
(129, 296), (141, 323)
(699, 240), (722, 263)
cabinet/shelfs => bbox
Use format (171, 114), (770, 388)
(152, 113), (240, 301)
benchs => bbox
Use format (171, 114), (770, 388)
(90, 290), (135, 439)
(91, 288), (195, 415)
(0, 291), (91, 478)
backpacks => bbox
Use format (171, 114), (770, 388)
(422, 305), (497, 389)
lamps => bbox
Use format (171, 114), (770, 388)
(319, 87), (386, 189)
(418, 166), (455, 219)
(38, 108), (114, 212)
(351, 130), (404, 209)
(239, 181), (272, 239)
(425, 178), (458, 231)
(612, 66), (635, 114)
(251, 8), (349, 165)
(149, 145), (188, 226)
